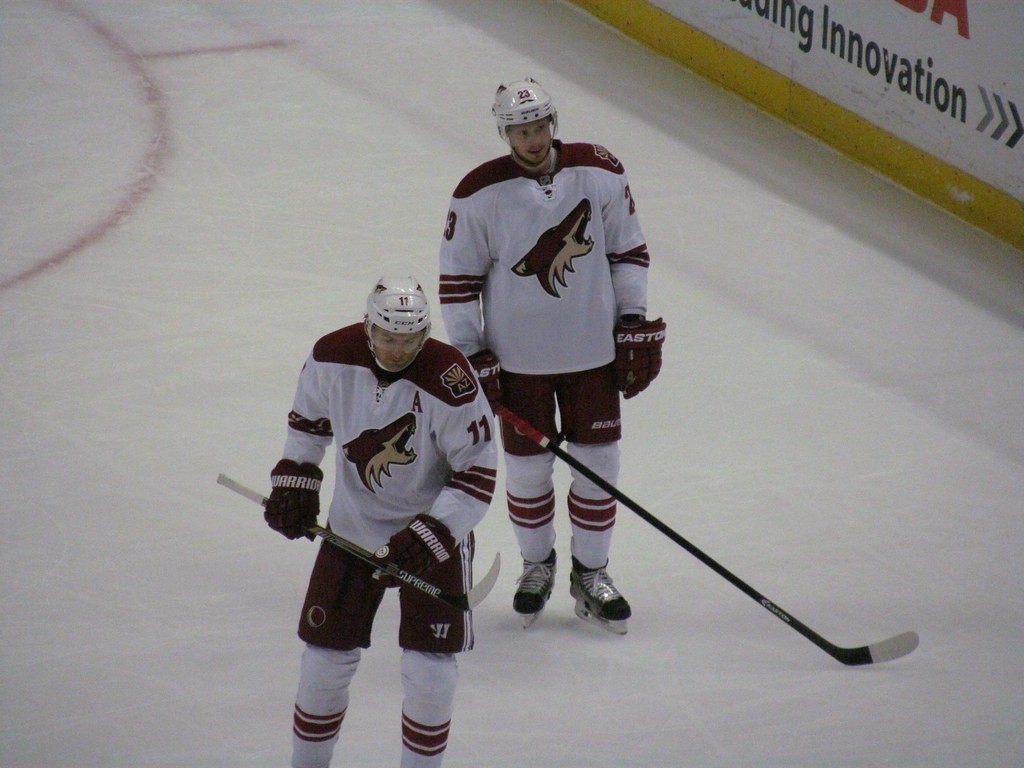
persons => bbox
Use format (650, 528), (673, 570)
(263, 279), (497, 768)
(439, 80), (665, 634)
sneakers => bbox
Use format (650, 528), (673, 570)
(514, 547), (557, 628)
(570, 553), (632, 634)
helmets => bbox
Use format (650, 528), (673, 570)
(364, 274), (432, 372)
(493, 77), (557, 147)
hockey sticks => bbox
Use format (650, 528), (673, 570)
(215, 469), (501, 611)
(494, 400), (922, 669)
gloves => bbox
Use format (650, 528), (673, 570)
(470, 352), (503, 402)
(371, 513), (457, 587)
(611, 317), (666, 400)
(264, 459), (324, 542)
